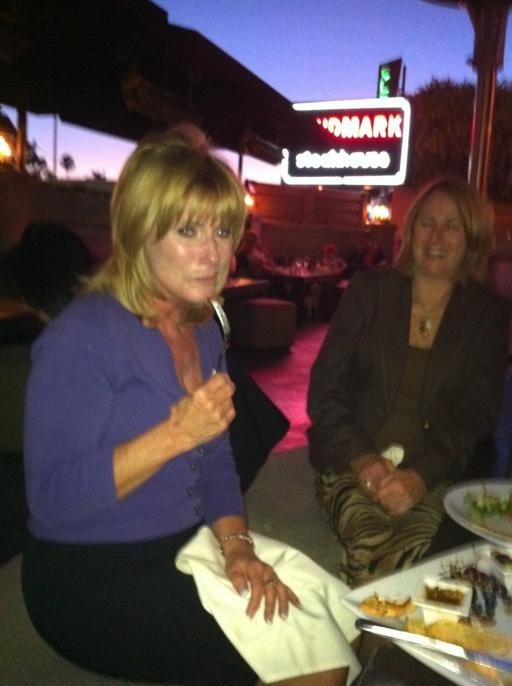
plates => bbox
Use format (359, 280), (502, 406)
(442, 480), (512, 548)
(344, 543), (512, 686)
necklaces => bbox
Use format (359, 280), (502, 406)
(411, 278), (447, 339)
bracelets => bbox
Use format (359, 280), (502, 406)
(220, 534), (256, 556)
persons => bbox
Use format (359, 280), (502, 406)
(307, 179), (512, 587)
(236, 232), (388, 314)
(23, 131), (365, 686)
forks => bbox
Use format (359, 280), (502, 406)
(207, 297), (232, 380)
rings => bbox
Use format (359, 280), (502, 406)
(265, 579), (279, 588)
(366, 481), (371, 488)
